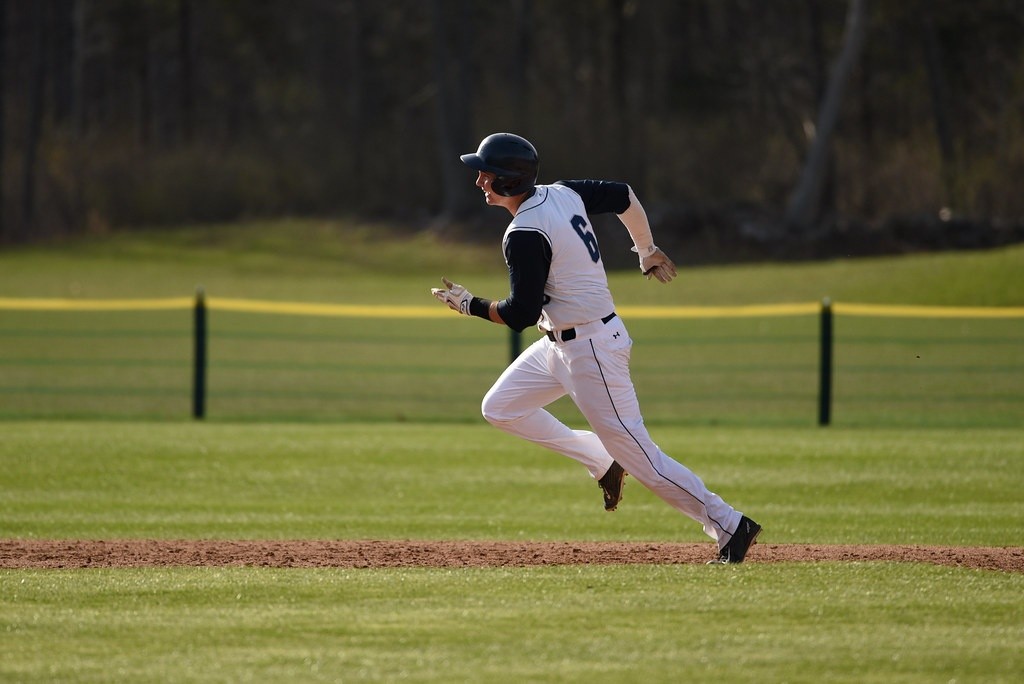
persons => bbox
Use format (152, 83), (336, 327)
(430, 133), (766, 564)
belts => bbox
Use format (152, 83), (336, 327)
(547, 312), (616, 342)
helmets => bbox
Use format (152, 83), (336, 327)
(460, 133), (540, 196)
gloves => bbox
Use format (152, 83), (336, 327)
(640, 247), (678, 283)
(430, 277), (474, 316)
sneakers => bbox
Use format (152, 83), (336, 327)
(597, 460), (629, 512)
(718, 516), (762, 565)
